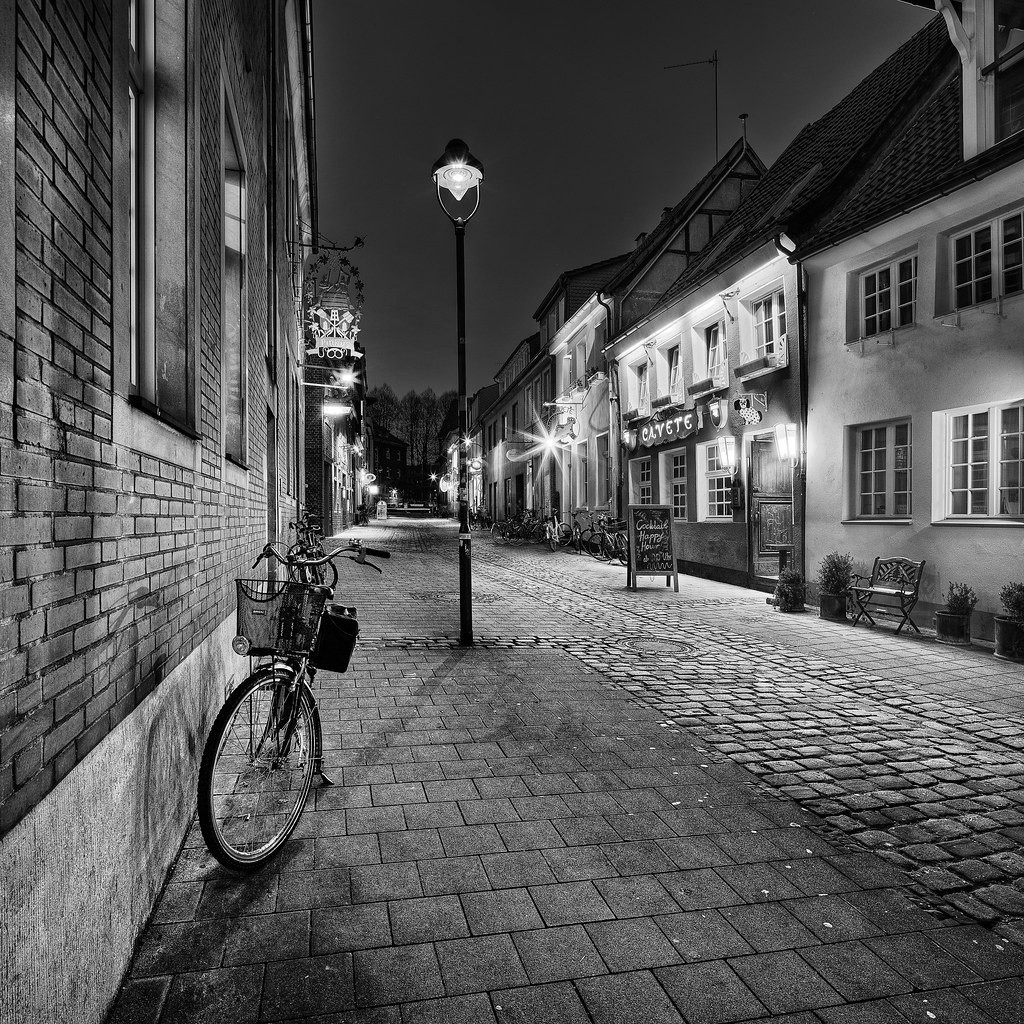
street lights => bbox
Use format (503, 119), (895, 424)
(428, 134), (480, 645)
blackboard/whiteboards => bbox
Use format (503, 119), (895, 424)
(628, 503), (679, 577)
(376, 501), (388, 519)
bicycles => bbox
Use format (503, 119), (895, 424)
(357, 503), (370, 527)
(286, 510), (339, 590)
(469, 499), (629, 567)
(197, 542), (390, 875)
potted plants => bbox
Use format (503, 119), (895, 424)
(994, 581), (1024, 658)
(569, 379), (583, 398)
(814, 551), (855, 620)
(934, 580), (980, 642)
(772, 564), (815, 614)
(586, 365), (605, 386)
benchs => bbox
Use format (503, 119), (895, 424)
(847, 556), (926, 634)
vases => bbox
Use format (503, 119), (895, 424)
(555, 396), (569, 407)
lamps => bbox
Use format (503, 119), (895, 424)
(715, 435), (741, 476)
(773, 423), (800, 468)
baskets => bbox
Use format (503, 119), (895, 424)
(234, 578), (327, 648)
(608, 521), (627, 532)
